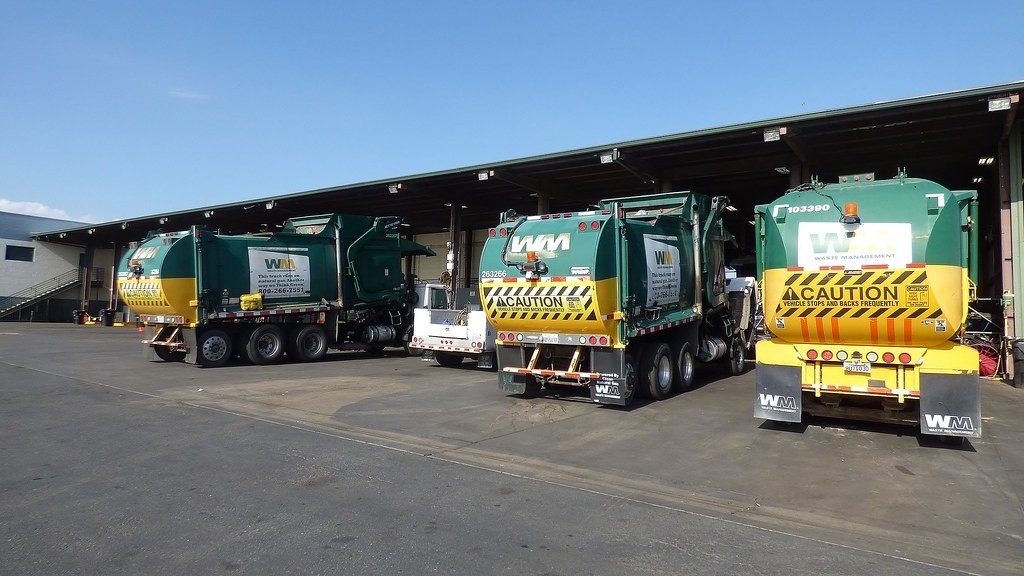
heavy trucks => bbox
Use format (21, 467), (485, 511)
(753, 164), (983, 442)
(116, 213), (437, 367)
(479, 188), (760, 410)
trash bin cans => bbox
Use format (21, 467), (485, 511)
(74, 310), (86, 324)
(100, 309), (115, 326)
